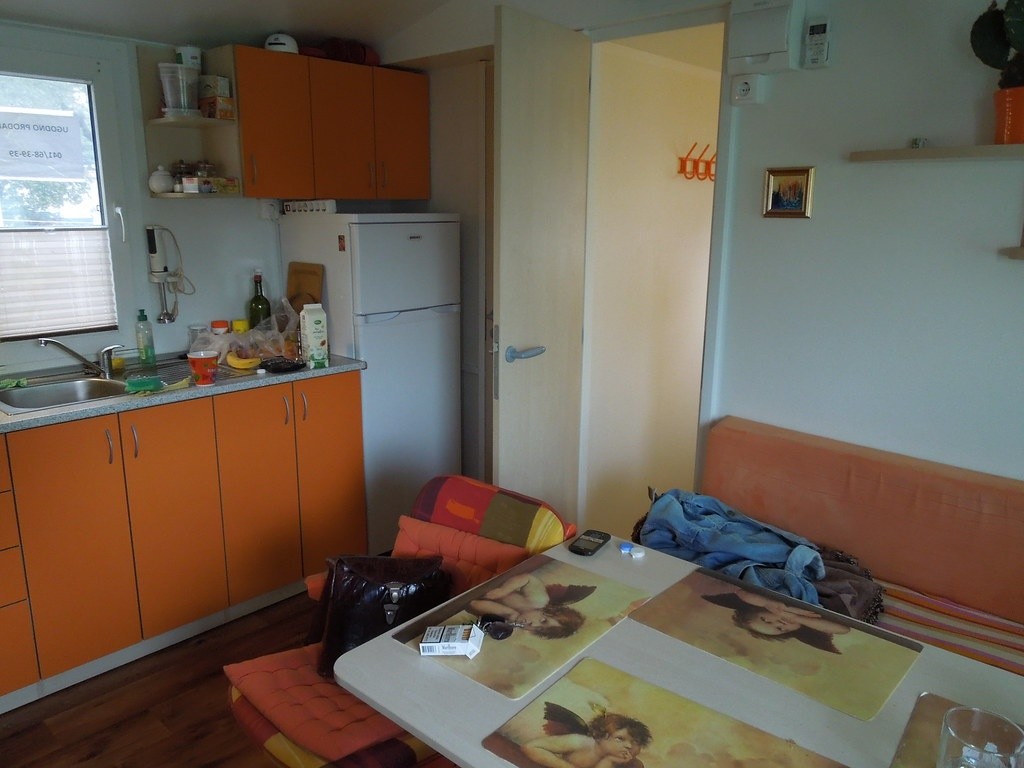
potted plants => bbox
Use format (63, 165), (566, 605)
(971, 0), (1023, 144)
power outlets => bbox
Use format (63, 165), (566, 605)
(731, 74), (768, 106)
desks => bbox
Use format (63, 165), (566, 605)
(334, 535), (1024, 768)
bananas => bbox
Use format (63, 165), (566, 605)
(226, 351), (263, 368)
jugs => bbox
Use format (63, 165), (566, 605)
(149, 165), (174, 193)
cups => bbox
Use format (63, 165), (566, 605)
(187, 350), (219, 387)
(157, 63), (201, 109)
(935, 706), (1024, 768)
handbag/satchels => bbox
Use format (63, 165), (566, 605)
(313, 559), (456, 678)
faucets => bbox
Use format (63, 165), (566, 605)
(38, 336), (124, 380)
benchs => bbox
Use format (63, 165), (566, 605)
(701, 416), (1024, 678)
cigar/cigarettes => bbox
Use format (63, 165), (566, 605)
(462, 629), (471, 640)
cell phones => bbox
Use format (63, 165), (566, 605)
(568, 529), (611, 556)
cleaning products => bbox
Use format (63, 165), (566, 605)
(135, 308), (156, 364)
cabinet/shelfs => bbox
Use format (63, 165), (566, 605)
(137, 44), (245, 199)
(849, 145), (1024, 260)
(6, 396), (230, 695)
(0, 432), (42, 716)
(309, 55), (430, 200)
(202, 44), (315, 200)
(213, 369), (369, 620)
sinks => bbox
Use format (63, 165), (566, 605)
(1, 378), (139, 416)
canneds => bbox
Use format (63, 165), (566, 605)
(211, 320), (230, 335)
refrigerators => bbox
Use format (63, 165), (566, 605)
(278, 213), (462, 560)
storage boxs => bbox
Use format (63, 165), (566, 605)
(199, 75), (235, 120)
(182, 177), (238, 193)
(419, 624), (485, 659)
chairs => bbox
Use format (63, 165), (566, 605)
(224, 475), (578, 768)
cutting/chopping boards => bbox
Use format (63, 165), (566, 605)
(285, 262), (323, 330)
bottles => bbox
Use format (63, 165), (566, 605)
(211, 320), (229, 335)
(232, 320), (249, 335)
(250, 274), (270, 334)
(174, 158), (215, 193)
(188, 325), (208, 348)
(135, 308), (156, 364)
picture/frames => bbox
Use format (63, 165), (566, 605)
(762, 166), (816, 218)
